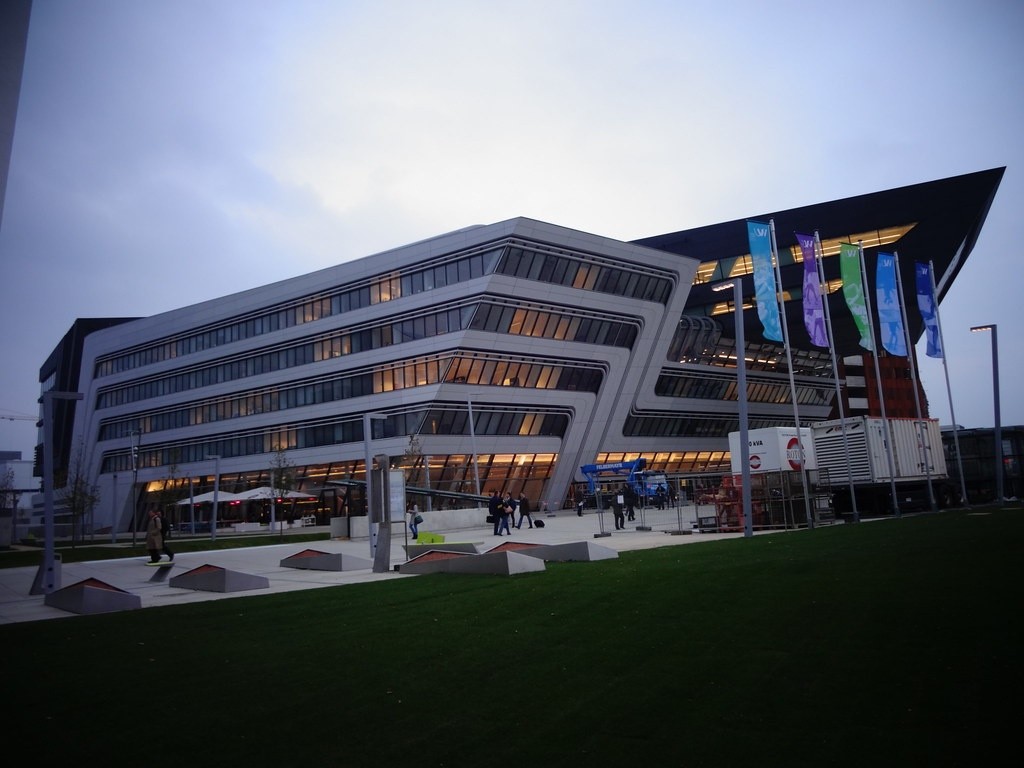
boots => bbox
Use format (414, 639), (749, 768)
(628, 515), (631, 520)
(632, 515), (635, 520)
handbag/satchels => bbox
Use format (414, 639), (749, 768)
(414, 515), (423, 524)
(578, 501), (584, 506)
(510, 500), (517, 511)
(618, 495), (624, 504)
(502, 505), (513, 513)
(487, 516), (495, 523)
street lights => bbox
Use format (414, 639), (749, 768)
(467, 391), (486, 508)
(131, 430), (138, 548)
(712, 277), (753, 537)
(42, 391), (84, 594)
(363, 413), (388, 559)
(424, 455), (435, 511)
(205, 455), (221, 541)
(970, 324), (1004, 506)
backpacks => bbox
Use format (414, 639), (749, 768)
(154, 517), (172, 537)
(671, 491), (676, 496)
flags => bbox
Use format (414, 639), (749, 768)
(745, 220), (944, 358)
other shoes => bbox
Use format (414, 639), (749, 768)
(494, 533), (498, 535)
(155, 556), (161, 562)
(667, 508), (669, 510)
(662, 508), (664, 510)
(620, 527), (625, 529)
(616, 527), (620, 529)
(415, 535), (417, 538)
(148, 561), (156, 563)
(507, 532), (511, 534)
(671, 508), (674, 510)
(529, 526), (533, 528)
(168, 552), (174, 561)
(658, 508), (660, 510)
(413, 536), (415, 539)
(515, 526), (520, 529)
(498, 533), (503, 536)
(512, 525), (515, 527)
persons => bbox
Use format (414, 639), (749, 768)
(654, 483), (665, 510)
(155, 511), (174, 562)
(146, 510), (163, 563)
(575, 486), (584, 516)
(407, 501), (420, 540)
(666, 482), (675, 510)
(514, 491), (533, 529)
(489, 491), (516, 536)
(611, 491), (625, 530)
(622, 483), (636, 521)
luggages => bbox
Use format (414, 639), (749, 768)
(529, 513), (545, 528)
